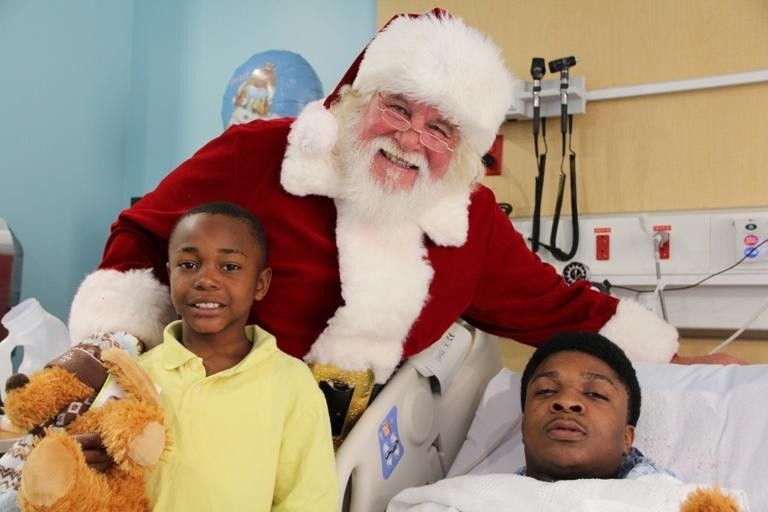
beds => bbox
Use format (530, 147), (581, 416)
(335, 317), (768, 512)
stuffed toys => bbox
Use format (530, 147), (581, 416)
(0, 267), (170, 511)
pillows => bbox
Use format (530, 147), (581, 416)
(441, 362), (768, 512)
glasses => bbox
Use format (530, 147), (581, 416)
(373, 96), (457, 155)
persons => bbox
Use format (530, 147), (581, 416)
(73, 7), (745, 422)
(70, 200), (343, 512)
(509, 330), (683, 485)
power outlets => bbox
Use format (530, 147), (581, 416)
(580, 213), (645, 275)
(645, 215), (712, 277)
